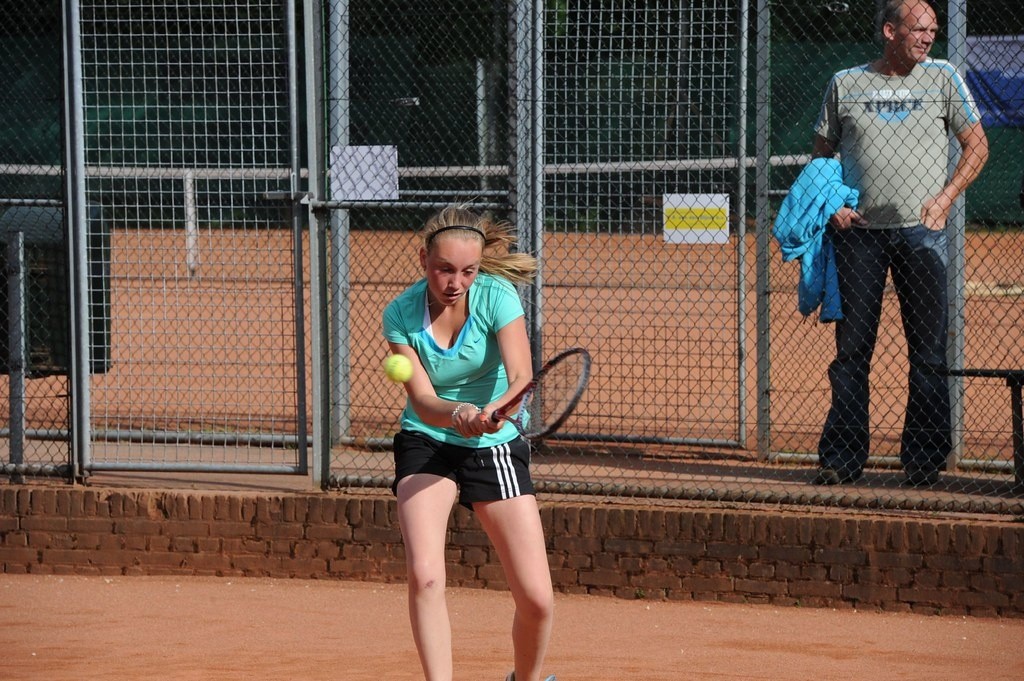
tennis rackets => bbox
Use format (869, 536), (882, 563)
(484, 347), (592, 441)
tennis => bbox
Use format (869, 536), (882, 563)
(385, 354), (414, 384)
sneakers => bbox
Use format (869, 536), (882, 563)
(908, 470), (946, 491)
(817, 467), (862, 484)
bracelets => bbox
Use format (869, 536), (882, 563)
(450, 400), (482, 427)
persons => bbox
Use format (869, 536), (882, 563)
(383, 204), (557, 680)
(808, 0), (989, 490)
